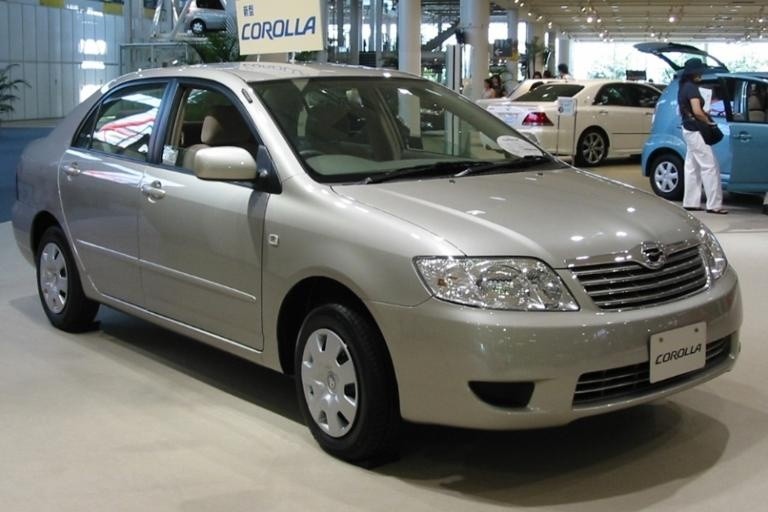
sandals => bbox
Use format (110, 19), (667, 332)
(683, 207), (728, 214)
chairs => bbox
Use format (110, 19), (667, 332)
(748, 95), (768, 122)
(183, 87), (375, 175)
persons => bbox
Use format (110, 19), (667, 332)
(481, 78), (496, 99)
(532, 71), (542, 78)
(544, 70), (551, 78)
(678, 57), (729, 214)
(558, 63), (573, 79)
(491, 75), (503, 98)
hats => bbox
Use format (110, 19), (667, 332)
(682, 58), (709, 77)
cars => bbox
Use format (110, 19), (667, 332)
(486, 78), (664, 168)
(183, 0), (228, 34)
(633, 43), (768, 201)
(10, 63), (744, 467)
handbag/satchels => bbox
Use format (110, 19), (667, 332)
(695, 116), (724, 145)
